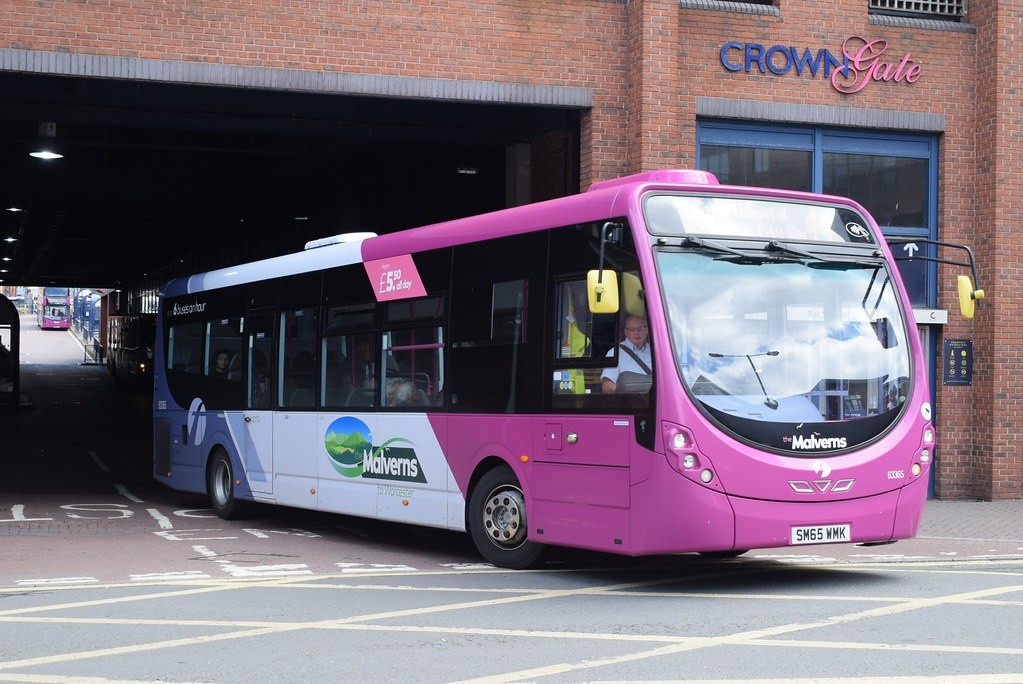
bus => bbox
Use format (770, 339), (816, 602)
(37, 287), (71, 329)
(151, 167), (985, 570)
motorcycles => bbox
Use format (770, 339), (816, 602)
(0, 294), (20, 416)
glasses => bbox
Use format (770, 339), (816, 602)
(625, 326), (645, 333)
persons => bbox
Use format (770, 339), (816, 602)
(213, 333), (430, 408)
(600, 315), (653, 393)
